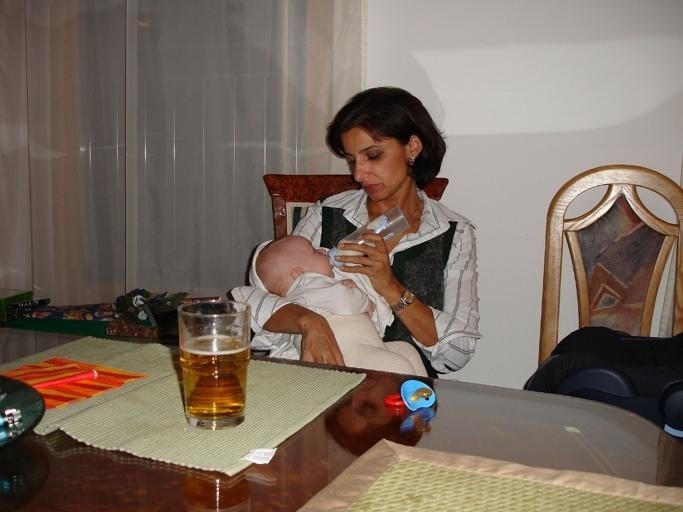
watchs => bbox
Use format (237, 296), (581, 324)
(388, 288), (415, 314)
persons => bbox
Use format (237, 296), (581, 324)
(254, 234), (431, 377)
(231, 86), (481, 380)
(324, 370), (438, 457)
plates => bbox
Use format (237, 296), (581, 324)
(0, 375), (46, 450)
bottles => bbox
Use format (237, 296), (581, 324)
(327, 206), (410, 268)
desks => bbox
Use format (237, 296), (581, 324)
(0, 328), (683, 512)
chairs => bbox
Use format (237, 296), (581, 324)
(538, 163), (683, 365)
(263, 174), (449, 240)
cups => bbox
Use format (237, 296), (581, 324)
(177, 300), (251, 430)
